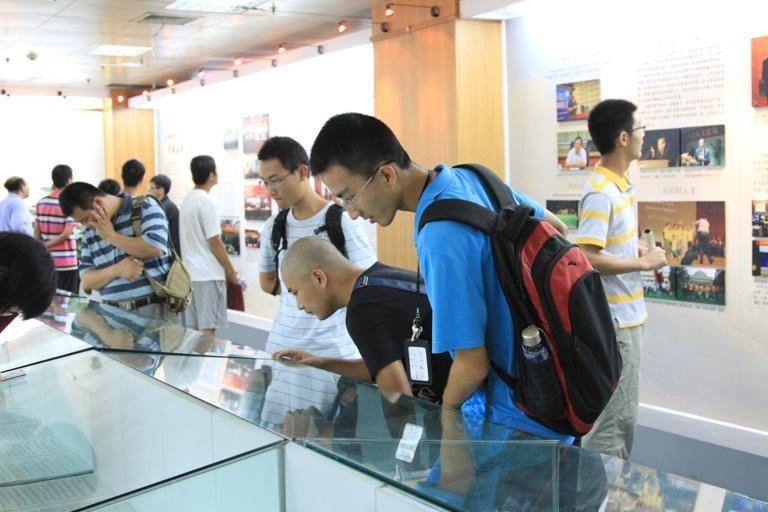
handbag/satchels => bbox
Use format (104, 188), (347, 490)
(135, 320), (187, 352)
(132, 194), (193, 312)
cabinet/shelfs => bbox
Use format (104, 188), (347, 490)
(0, 286), (768, 512)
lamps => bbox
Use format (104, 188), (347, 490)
(385, 3), (395, 18)
(116, 93), (125, 106)
(338, 21), (348, 33)
(279, 44), (287, 55)
(167, 78), (174, 87)
(234, 56), (241, 67)
(197, 68), (204, 80)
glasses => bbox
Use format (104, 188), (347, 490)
(259, 163), (304, 189)
(342, 161), (396, 213)
(623, 123), (649, 134)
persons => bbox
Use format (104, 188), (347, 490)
(662, 222), (695, 260)
(1, 231), (58, 333)
(0, 175), (35, 236)
(119, 159), (145, 198)
(575, 99), (669, 461)
(310, 112), (575, 445)
(565, 87), (589, 117)
(35, 164), (80, 295)
(178, 155), (237, 334)
(149, 175), (182, 256)
(694, 138), (711, 166)
(99, 179), (120, 196)
(59, 183), (174, 321)
(692, 216), (716, 266)
(272, 237), (452, 405)
(649, 138), (677, 167)
(566, 137), (588, 170)
(257, 137), (377, 360)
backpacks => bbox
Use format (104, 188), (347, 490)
(419, 163), (623, 437)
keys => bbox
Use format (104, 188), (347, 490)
(410, 318), (423, 342)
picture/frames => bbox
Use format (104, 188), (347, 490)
(556, 130), (600, 175)
(245, 226), (261, 248)
(751, 35), (768, 108)
(219, 216), (240, 255)
(555, 79), (602, 123)
(752, 200), (768, 278)
(638, 201), (725, 307)
(244, 184), (272, 221)
(637, 128), (679, 168)
(680, 125), (726, 168)
(546, 199), (579, 230)
(242, 114), (269, 179)
